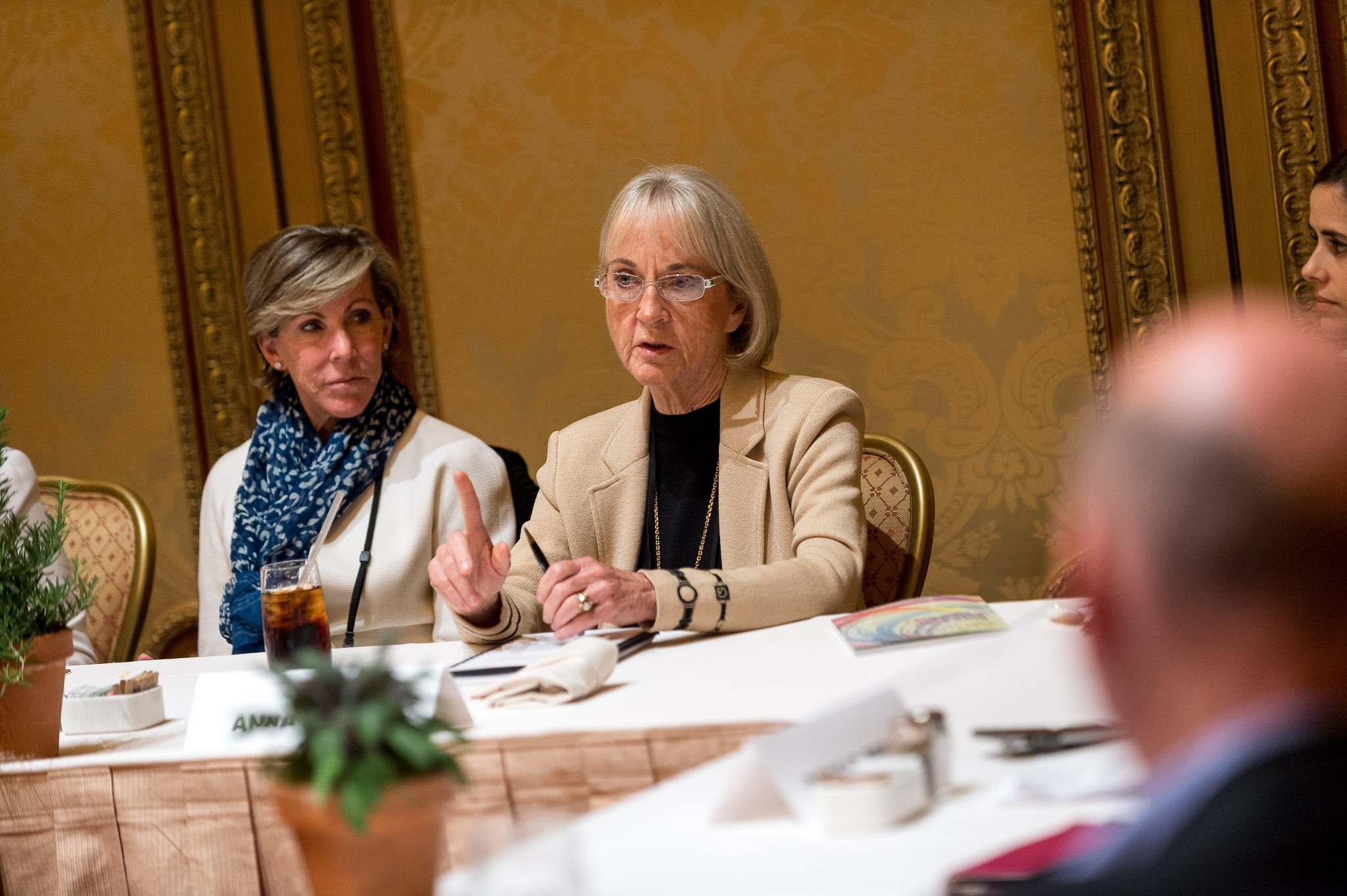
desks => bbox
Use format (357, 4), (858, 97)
(50, 590), (1155, 896)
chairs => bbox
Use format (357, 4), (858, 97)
(858, 430), (935, 606)
(38, 476), (163, 666)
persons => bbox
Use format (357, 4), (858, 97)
(0, 445), (97, 667)
(1302, 154), (1347, 357)
(137, 221), (519, 662)
(1027, 299), (1347, 894)
(427, 163), (866, 646)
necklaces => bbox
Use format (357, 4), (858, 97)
(649, 465), (716, 579)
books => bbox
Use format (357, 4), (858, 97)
(831, 593), (1011, 656)
(944, 823), (1137, 893)
(446, 629), (661, 676)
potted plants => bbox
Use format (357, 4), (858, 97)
(0, 407), (97, 760)
(259, 641), (466, 896)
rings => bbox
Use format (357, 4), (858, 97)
(578, 590), (593, 612)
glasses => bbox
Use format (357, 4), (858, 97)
(594, 272), (727, 302)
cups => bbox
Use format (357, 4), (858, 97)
(260, 560), (331, 683)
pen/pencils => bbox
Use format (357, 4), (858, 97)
(522, 526), (550, 577)
